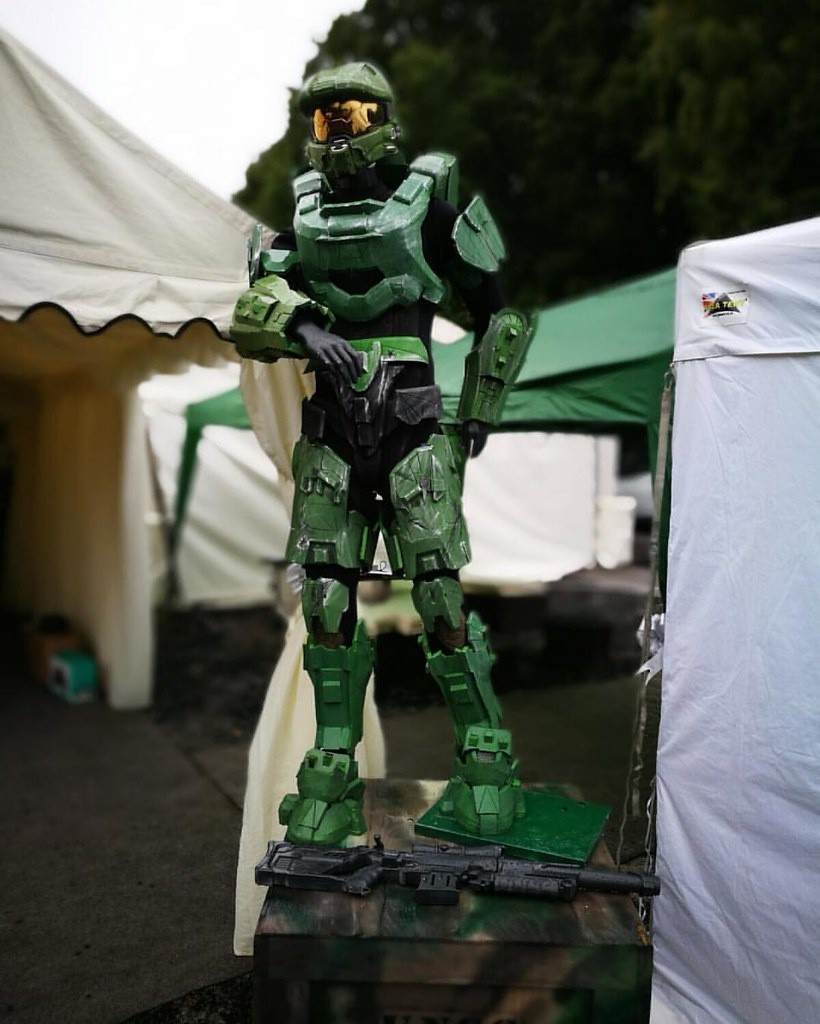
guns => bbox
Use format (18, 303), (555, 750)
(251, 832), (666, 909)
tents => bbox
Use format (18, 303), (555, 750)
(0, 29), (819, 1024)
